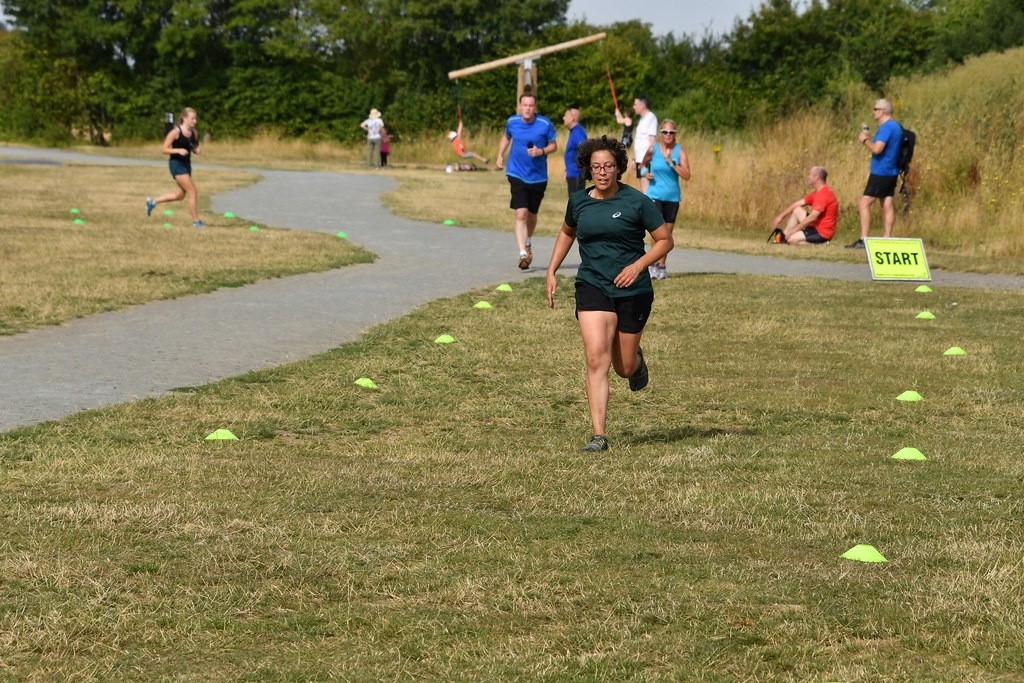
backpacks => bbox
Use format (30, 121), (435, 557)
(896, 123), (916, 168)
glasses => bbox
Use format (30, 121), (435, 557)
(873, 106), (883, 111)
(660, 129), (677, 135)
(588, 163), (618, 173)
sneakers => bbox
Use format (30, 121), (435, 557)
(648, 262), (658, 279)
(630, 346), (648, 390)
(659, 265), (666, 279)
(582, 435), (608, 451)
(845, 240), (864, 248)
(526, 243), (532, 264)
(519, 254), (529, 270)
(146, 196), (155, 216)
(193, 219), (206, 228)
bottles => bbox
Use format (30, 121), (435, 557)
(446, 162), (459, 173)
(862, 125), (870, 134)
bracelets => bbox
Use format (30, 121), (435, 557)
(541, 148), (544, 156)
(863, 138), (870, 144)
(640, 167), (649, 176)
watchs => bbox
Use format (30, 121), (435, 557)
(670, 160), (676, 167)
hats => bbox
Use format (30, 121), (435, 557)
(369, 108), (381, 119)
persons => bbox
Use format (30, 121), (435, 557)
(360, 108), (385, 167)
(615, 107), (633, 155)
(845, 98), (905, 249)
(562, 109), (588, 200)
(146, 108), (207, 229)
(546, 134), (674, 452)
(380, 128), (394, 167)
(632, 97), (658, 195)
(448, 120), (491, 164)
(496, 92), (557, 270)
(772, 166), (838, 244)
(639, 119), (691, 280)
